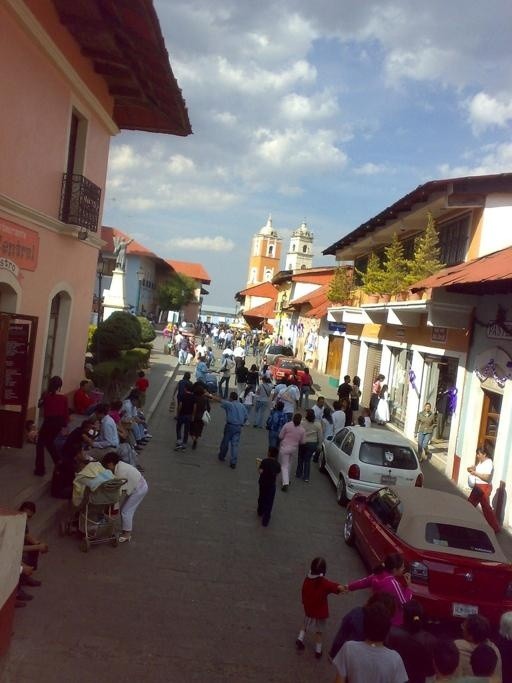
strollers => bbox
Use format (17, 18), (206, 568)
(58, 471), (127, 552)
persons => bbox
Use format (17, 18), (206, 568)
(66, 421), (92, 452)
(350, 376), (361, 425)
(343, 554), (413, 626)
(83, 417), (101, 450)
(89, 418), (145, 472)
(34, 376), (68, 476)
(119, 394), (150, 446)
(133, 388), (152, 439)
(337, 375), (353, 409)
(369, 374), (385, 422)
(106, 401), (143, 455)
(314, 408), (335, 462)
(261, 364), (273, 381)
(137, 372), (148, 415)
(455, 614), (502, 683)
(385, 601), (438, 683)
(196, 356), (213, 384)
(430, 640), (466, 683)
(468, 448), (501, 534)
(86, 405), (119, 457)
(267, 402), (288, 447)
(253, 376), (273, 428)
(74, 380), (100, 415)
(279, 413), (305, 491)
(345, 408), (356, 427)
(173, 372), (193, 415)
(436, 388), (448, 440)
(312, 396), (325, 419)
(329, 593), (395, 663)
(245, 365), (259, 406)
(272, 379), (286, 402)
(102, 453), (147, 542)
(331, 402), (346, 435)
(288, 368), (302, 408)
(174, 385), (196, 453)
(414, 403), (437, 462)
(16, 561), (34, 607)
(278, 381), (299, 422)
(17, 502), (49, 586)
(257, 447), (282, 526)
(463, 645), (499, 683)
(333, 610), (408, 683)
(190, 385), (211, 450)
(167, 322), (293, 366)
(300, 368), (313, 409)
(374, 385), (390, 425)
(297, 401), (298, 401)
(355, 417), (366, 427)
(215, 353), (235, 399)
(73, 453), (116, 505)
(235, 360), (249, 403)
(295, 557), (345, 659)
(296, 409), (323, 482)
(204, 389), (248, 468)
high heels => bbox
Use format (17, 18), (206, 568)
(119, 531), (125, 535)
(118, 535), (131, 542)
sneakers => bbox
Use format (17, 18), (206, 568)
(18, 589), (34, 601)
(281, 485), (289, 492)
(295, 638), (303, 649)
(19, 575), (41, 587)
(314, 651), (322, 660)
(181, 445), (187, 453)
(174, 442), (182, 450)
(218, 453), (224, 462)
(230, 463), (237, 469)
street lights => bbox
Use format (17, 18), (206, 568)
(97, 253), (104, 363)
(275, 292), (287, 343)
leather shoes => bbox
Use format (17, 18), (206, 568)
(135, 445), (142, 451)
(143, 434), (152, 438)
(137, 441), (145, 445)
(141, 438), (151, 443)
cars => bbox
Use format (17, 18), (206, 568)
(269, 356), (313, 388)
(178, 322), (195, 336)
(318, 425), (425, 505)
(343, 484), (512, 631)
(259, 343), (294, 373)
